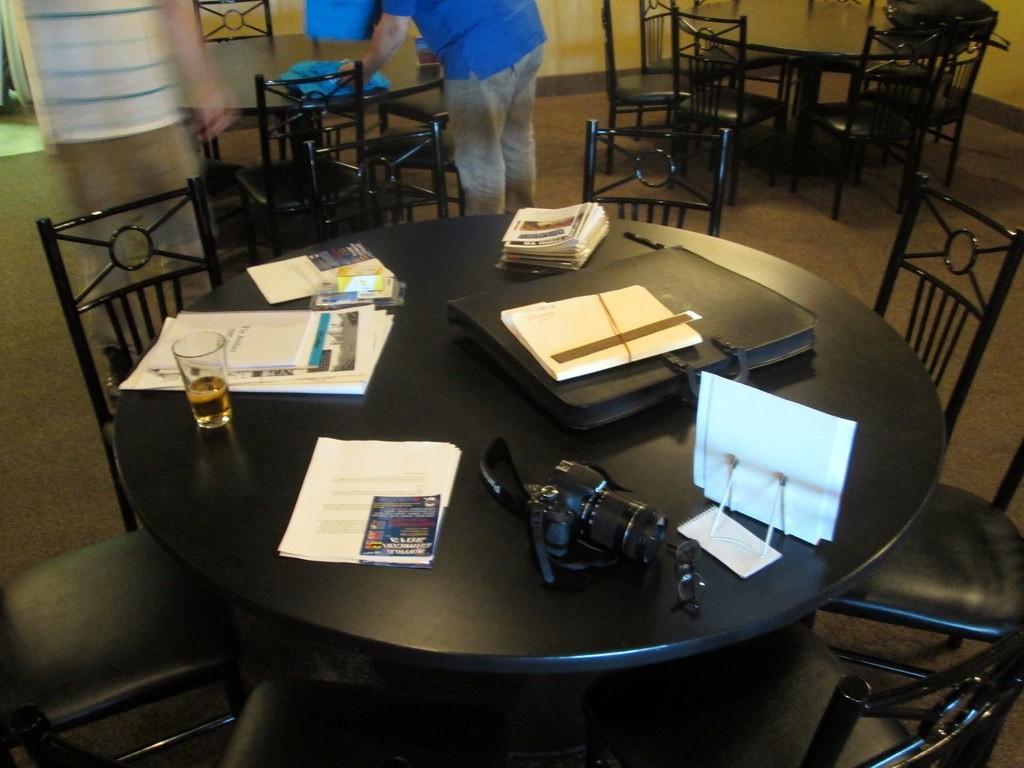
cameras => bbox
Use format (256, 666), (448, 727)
(525, 459), (668, 592)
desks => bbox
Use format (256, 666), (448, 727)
(178, 32), (445, 167)
(115, 205), (951, 674)
(645, 4), (1001, 223)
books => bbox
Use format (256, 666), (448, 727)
(150, 309), (314, 368)
(501, 202), (608, 272)
(676, 504), (782, 580)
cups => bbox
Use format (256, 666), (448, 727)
(170, 331), (234, 429)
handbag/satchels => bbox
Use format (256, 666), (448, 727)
(275, 59), (392, 100)
(445, 244), (821, 430)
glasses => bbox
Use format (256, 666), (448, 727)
(665, 540), (701, 616)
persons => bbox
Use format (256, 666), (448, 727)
(19, 0), (228, 400)
(337, 0), (545, 214)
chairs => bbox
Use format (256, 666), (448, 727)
(1, 3), (1024, 767)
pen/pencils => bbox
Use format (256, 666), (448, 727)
(623, 230), (665, 250)
